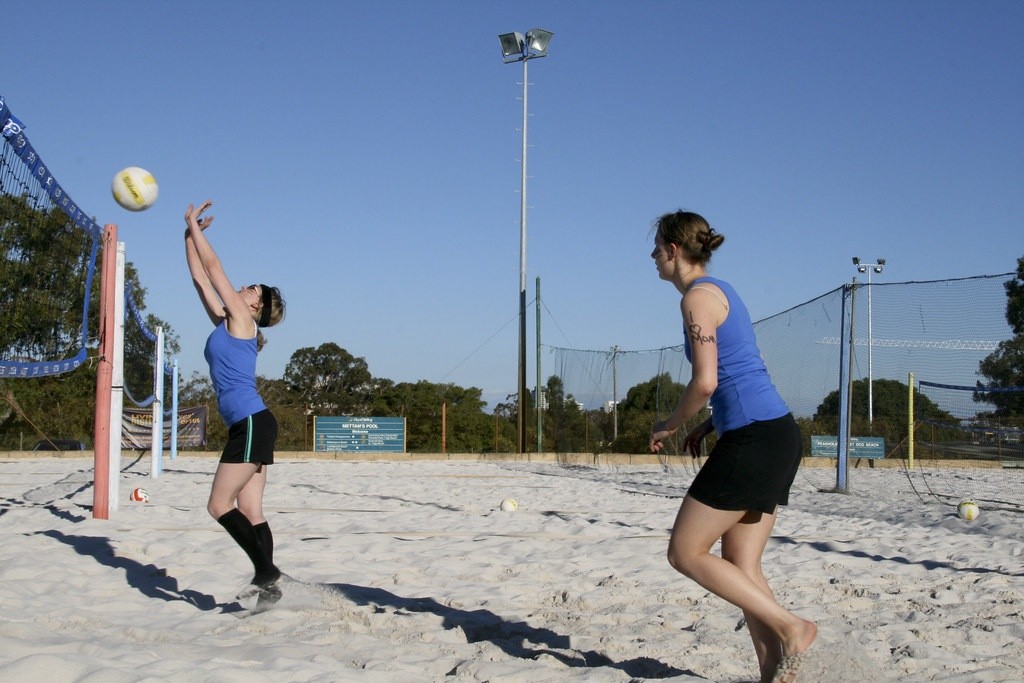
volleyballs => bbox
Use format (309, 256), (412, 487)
(111, 167), (159, 212)
(129, 486), (150, 504)
(500, 497), (521, 512)
(958, 499), (980, 521)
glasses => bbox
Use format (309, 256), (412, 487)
(249, 285), (260, 296)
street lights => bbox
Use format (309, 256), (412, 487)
(853, 255), (886, 432)
(496, 28), (556, 453)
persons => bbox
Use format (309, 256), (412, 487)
(185, 198), (286, 618)
(649, 213), (817, 682)
